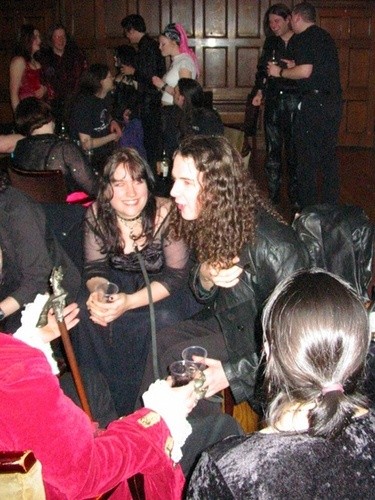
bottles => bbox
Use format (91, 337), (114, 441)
(271, 49), (278, 66)
(156, 148), (169, 179)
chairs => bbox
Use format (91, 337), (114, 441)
(6, 162), (68, 204)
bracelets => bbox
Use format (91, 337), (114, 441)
(0, 307), (5, 320)
(280, 67), (288, 78)
(162, 84), (168, 90)
(110, 119), (118, 127)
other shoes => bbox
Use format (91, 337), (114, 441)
(264, 191), (301, 216)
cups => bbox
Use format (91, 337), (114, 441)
(84, 138), (94, 155)
(169, 361), (198, 387)
(182, 346), (208, 371)
(95, 283), (119, 304)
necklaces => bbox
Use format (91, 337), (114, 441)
(114, 208), (145, 240)
(114, 208), (145, 222)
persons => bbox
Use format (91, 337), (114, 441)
(228, 4), (304, 211)
(121, 13), (166, 151)
(38, 23), (88, 136)
(70, 62), (124, 175)
(266, 2), (342, 214)
(9, 24), (55, 113)
(184, 268), (375, 500)
(0, 160), (81, 369)
(112, 44), (149, 163)
(13, 97), (100, 236)
(1, 236), (246, 500)
(66, 145), (191, 416)
(0, 133), (27, 153)
(169, 133), (375, 416)
(147, 78), (209, 179)
(152, 23), (202, 177)
